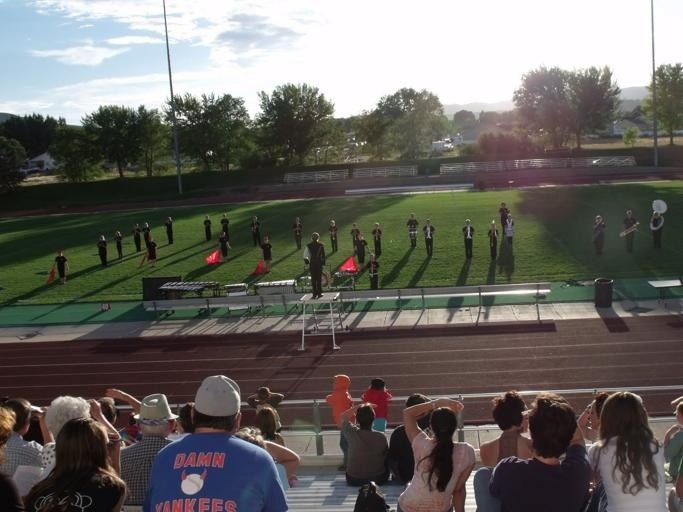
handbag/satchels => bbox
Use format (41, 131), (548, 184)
(353, 480), (389, 511)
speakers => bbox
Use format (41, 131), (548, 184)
(143, 277), (182, 301)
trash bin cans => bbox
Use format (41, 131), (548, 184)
(594, 278), (613, 308)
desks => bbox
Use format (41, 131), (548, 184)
(648, 279), (682, 305)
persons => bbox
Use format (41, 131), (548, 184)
(220, 213), (230, 236)
(326, 373), (683, 512)
(251, 216), (261, 246)
(488, 202), (514, 259)
(593, 215), (607, 253)
(164, 217), (173, 244)
(97, 235), (107, 265)
(131, 224), (142, 253)
(621, 210), (636, 253)
(142, 222), (152, 248)
(406, 213), (419, 247)
(423, 219), (435, 257)
(461, 219), (475, 259)
(1, 375), (300, 512)
(350, 222), (360, 248)
(649, 212), (664, 248)
(259, 235), (273, 273)
(114, 231), (124, 260)
(304, 232), (326, 298)
(219, 232), (229, 257)
(357, 234), (367, 264)
(146, 237), (157, 268)
(367, 253), (379, 291)
(53, 249), (69, 284)
(329, 220), (337, 252)
(372, 223), (381, 254)
(294, 216), (302, 248)
(204, 215), (211, 241)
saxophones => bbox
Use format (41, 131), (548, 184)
(620, 221), (640, 236)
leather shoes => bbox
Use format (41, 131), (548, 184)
(318, 294), (323, 297)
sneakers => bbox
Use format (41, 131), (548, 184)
(338, 464), (344, 470)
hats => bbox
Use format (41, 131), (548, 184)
(132, 393), (179, 425)
(193, 375), (240, 417)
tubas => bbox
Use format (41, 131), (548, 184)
(649, 200), (668, 231)
